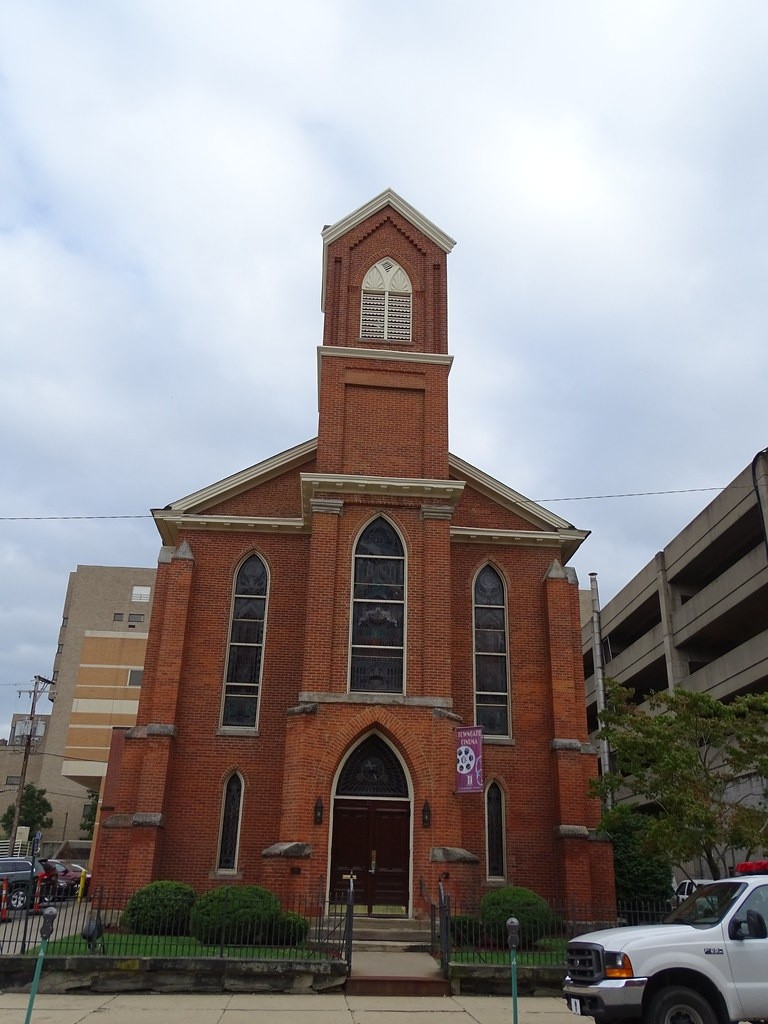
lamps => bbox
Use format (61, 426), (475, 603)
(422, 799), (431, 828)
(313, 796), (324, 824)
(81, 919), (102, 949)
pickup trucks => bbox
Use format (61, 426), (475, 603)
(666, 878), (717, 916)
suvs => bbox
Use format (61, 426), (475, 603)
(559, 859), (768, 1024)
(0, 855), (60, 910)
(42, 857), (90, 902)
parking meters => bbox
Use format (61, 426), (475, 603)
(505, 917), (522, 1024)
(22, 906), (59, 1024)
